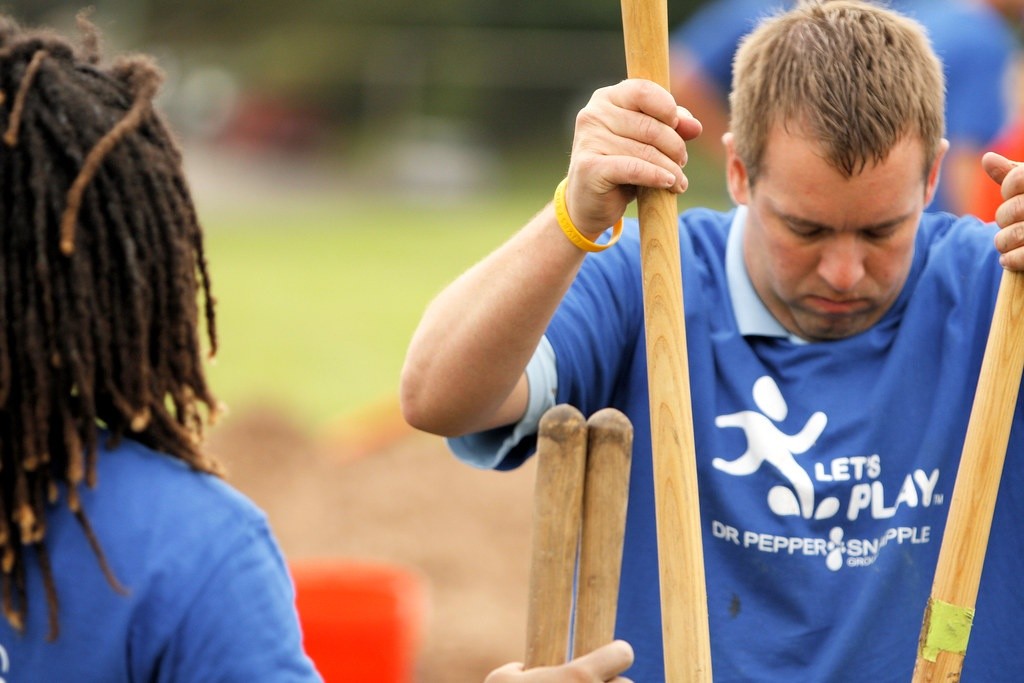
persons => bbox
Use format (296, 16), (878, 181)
(649, 1), (1023, 224)
(0, 10), (639, 683)
(397, 2), (1024, 682)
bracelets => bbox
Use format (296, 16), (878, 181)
(556, 170), (624, 253)
